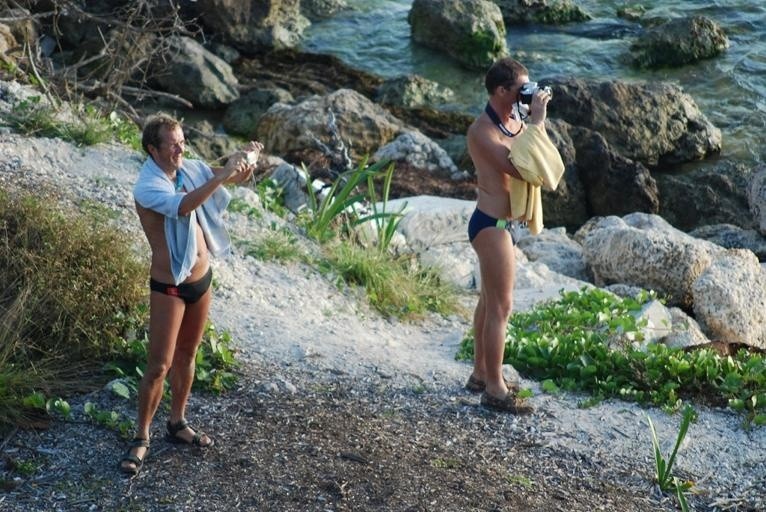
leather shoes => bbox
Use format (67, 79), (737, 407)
(465, 374), (520, 392)
(481, 390), (536, 415)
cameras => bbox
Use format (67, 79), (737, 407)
(244, 151), (258, 166)
(520, 82), (553, 104)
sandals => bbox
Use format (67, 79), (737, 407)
(164, 419), (214, 449)
(117, 438), (151, 474)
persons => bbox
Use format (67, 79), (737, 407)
(117, 112), (265, 475)
(467, 56), (553, 416)
